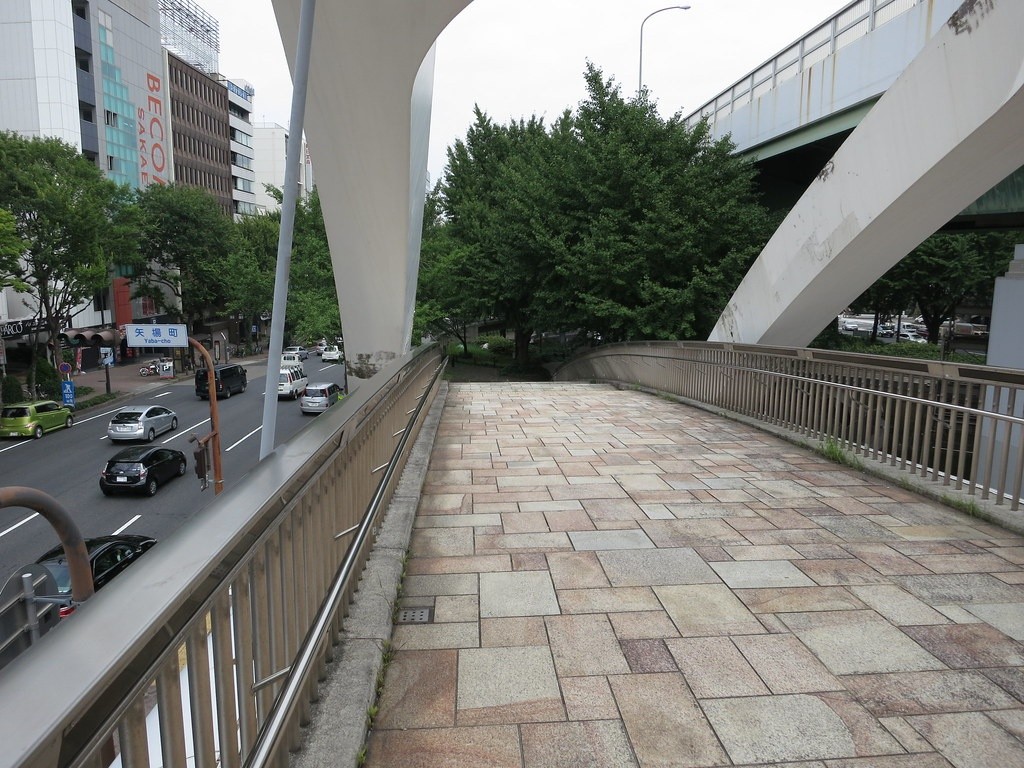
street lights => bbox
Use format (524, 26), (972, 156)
(636, 4), (693, 102)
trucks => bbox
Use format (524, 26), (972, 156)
(281, 353), (304, 370)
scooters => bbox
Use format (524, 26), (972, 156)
(138, 361), (160, 377)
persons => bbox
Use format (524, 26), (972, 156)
(116, 550), (123, 562)
(338, 389), (345, 400)
(188, 355), (205, 369)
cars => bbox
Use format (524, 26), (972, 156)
(842, 320), (858, 330)
(300, 382), (343, 413)
(870, 324), (895, 337)
(98, 445), (187, 497)
(915, 315), (925, 323)
(0, 400), (74, 440)
(283, 345), (309, 360)
(901, 333), (927, 344)
(26, 532), (160, 620)
(321, 345), (345, 362)
(107, 405), (179, 443)
(316, 342), (327, 355)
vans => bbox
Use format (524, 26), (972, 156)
(278, 365), (308, 400)
(195, 363), (248, 400)
(901, 322), (917, 335)
